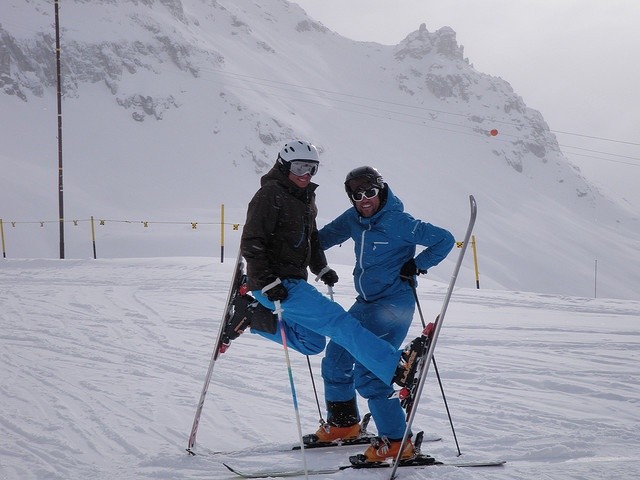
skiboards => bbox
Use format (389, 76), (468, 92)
(188, 195), (477, 478)
(186, 436), (506, 477)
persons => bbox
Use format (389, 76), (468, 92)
(221, 142), (433, 391)
(302, 166), (456, 463)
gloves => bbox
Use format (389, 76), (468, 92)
(321, 269), (338, 287)
(266, 276), (287, 301)
(400, 258), (428, 282)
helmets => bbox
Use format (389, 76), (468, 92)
(345, 166), (384, 203)
(279, 140), (320, 176)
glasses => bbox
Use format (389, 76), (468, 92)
(279, 156), (318, 176)
(352, 188), (380, 202)
(344, 173), (383, 192)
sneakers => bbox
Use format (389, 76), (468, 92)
(316, 423), (361, 442)
(393, 337), (427, 388)
(364, 438), (414, 463)
(224, 295), (260, 339)
